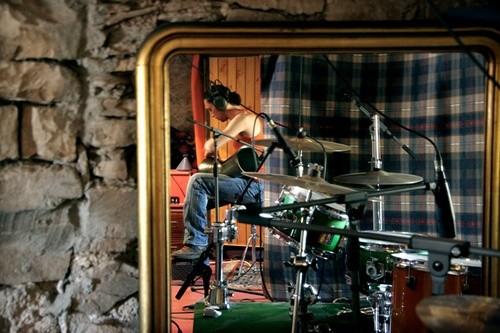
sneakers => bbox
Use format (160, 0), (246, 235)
(171, 244), (207, 260)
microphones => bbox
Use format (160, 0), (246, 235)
(433, 152), (457, 238)
(264, 115), (301, 168)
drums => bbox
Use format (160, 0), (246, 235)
(391, 252), (482, 333)
(347, 229), (423, 296)
(267, 186), (349, 258)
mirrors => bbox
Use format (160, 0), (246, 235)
(136, 22), (500, 333)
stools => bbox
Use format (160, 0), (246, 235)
(226, 201), (269, 289)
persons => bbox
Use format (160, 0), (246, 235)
(170, 85), (265, 261)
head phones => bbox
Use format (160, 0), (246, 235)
(208, 82), (228, 111)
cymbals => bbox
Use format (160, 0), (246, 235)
(253, 137), (352, 152)
(415, 293), (500, 333)
(333, 170), (423, 186)
(242, 171), (356, 195)
